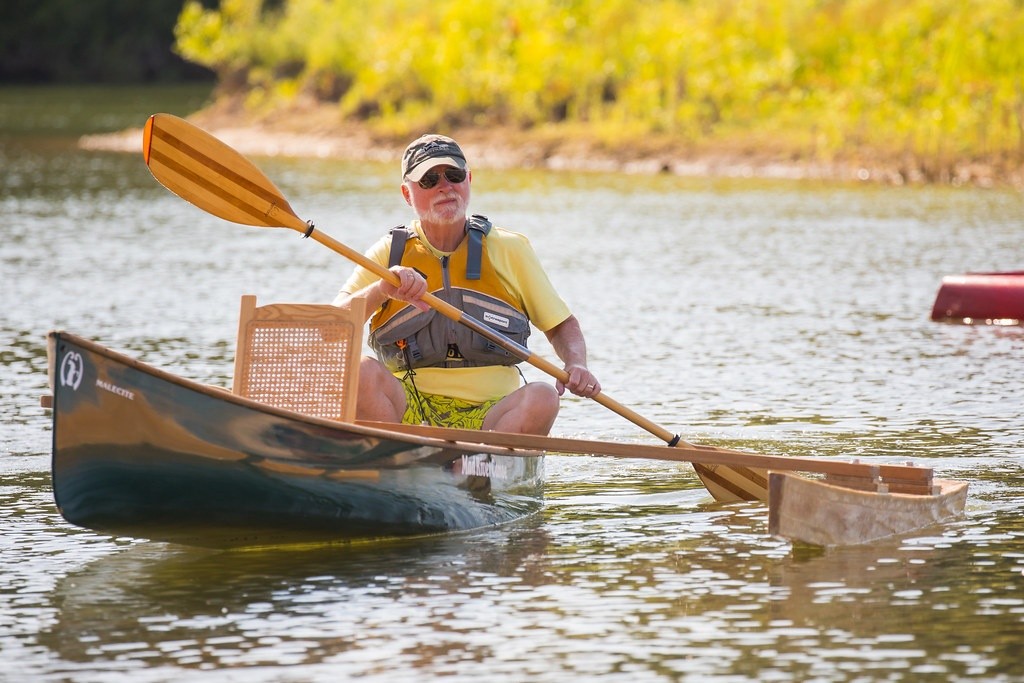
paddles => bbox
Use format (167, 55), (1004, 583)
(142, 113), (800, 506)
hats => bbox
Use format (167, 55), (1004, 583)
(402, 134), (467, 182)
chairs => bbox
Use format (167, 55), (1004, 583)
(230, 286), (369, 427)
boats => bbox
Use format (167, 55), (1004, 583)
(40, 330), (970, 551)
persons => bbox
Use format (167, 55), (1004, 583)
(318, 134), (601, 436)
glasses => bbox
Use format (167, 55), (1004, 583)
(417, 169), (467, 189)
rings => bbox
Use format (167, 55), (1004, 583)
(407, 273), (414, 277)
(586, 383), (595, 390)
